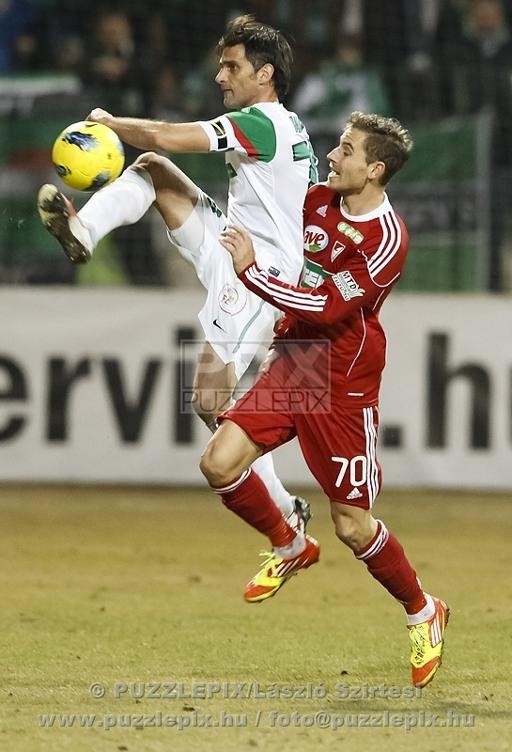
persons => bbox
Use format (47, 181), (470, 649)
(34, 14), (321, 537)
(0, 0), (511, 290)
(195, 110), (450, 688)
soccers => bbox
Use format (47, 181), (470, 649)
(53, 121), (125, 192)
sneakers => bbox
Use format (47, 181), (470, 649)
(242, 494), (321, 603)
(38, 183), (93, 264)
(406, 592), (450, 688)
